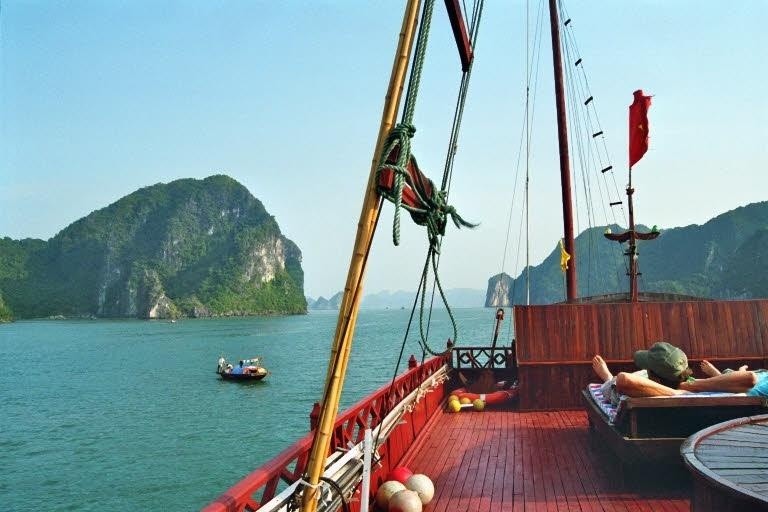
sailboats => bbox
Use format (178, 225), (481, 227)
(191, 2), (767, 511)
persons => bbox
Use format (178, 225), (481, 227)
(233, 361), (248, 374)
(700, 359), (768, 396)
(226, 364), (233, 370)
(592, 342), (756, 396)
(217, 356), (225, 373)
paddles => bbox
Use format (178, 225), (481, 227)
(481, 308), (504, 391)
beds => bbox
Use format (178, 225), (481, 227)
(579, 378), (767, 492)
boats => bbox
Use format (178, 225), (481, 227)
(214, 364), (267, 382)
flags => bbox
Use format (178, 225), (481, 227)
(560, 241), (570, 272)
(628, 89), (653, 167)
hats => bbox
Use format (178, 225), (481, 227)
(634, 342), (688, 381)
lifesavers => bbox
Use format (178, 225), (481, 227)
(450, 380), (519, 405)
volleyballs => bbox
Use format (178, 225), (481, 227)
(448, 395), (484, 411)
(379, 467), (435, 512)
